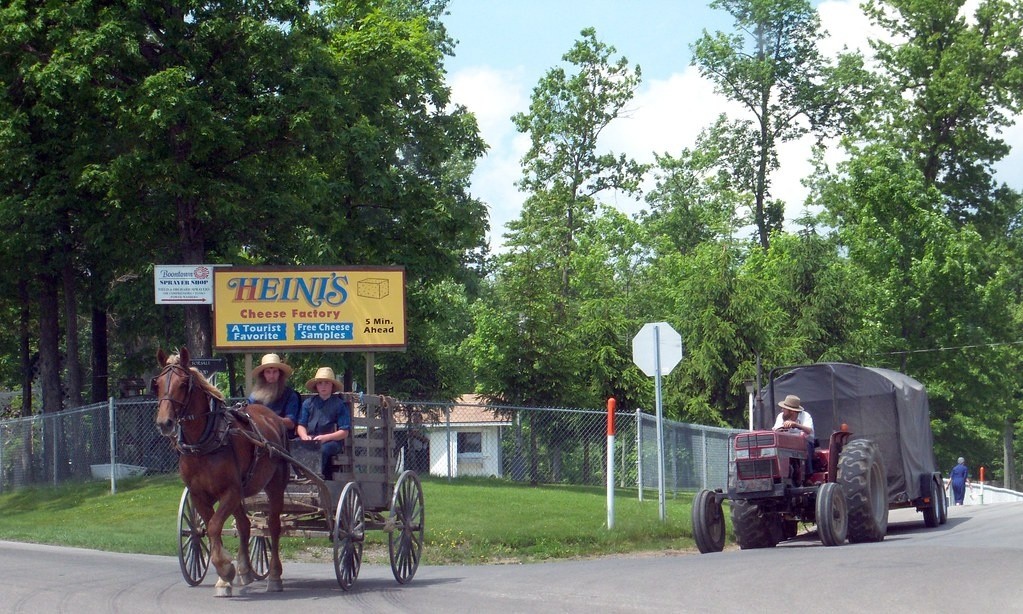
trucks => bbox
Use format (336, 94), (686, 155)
(690, 353), (947, 553)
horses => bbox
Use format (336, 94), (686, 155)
(156, 346), (302, 598)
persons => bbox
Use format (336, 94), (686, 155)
(289, 366), (352, 481)
(243, 354), (301, 441)
(770, 394), (816, 488)
(945, 456), (973, 506)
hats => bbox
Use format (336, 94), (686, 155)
(778, 395), (805, 412)
(305, 367), (342, 393)
(251, 354), (291, 379)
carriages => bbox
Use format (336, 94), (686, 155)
(148, 341), (424, 590)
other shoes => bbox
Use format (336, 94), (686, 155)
(804, 474), (813, 486)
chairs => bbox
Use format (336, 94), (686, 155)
(813, 436), (823, 464)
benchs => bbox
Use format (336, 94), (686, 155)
(288, 394), (351, 480)
